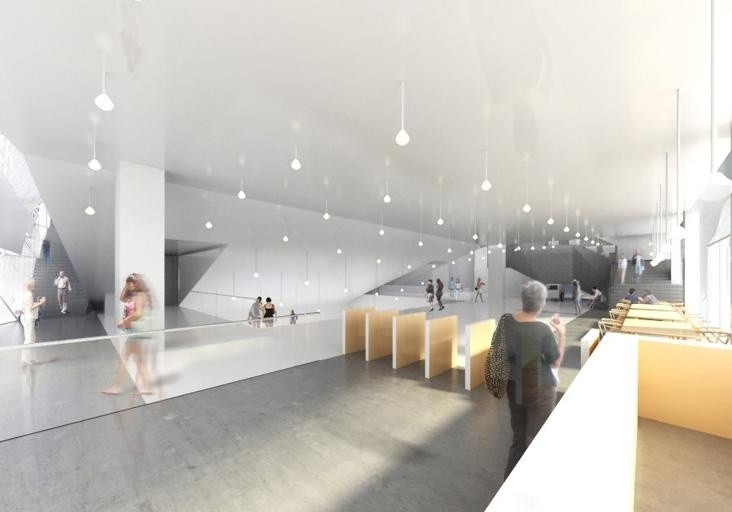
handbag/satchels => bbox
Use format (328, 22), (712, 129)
(483, 313), (512, 400)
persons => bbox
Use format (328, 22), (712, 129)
(42, 239), (51, 264)
(625, 288), (640, 304)
(290, 309), (297, 323)
(53, 271), (72, 314)
(638, 288), (658, 304)
(102, 273), (154, 393)
(632, 247), (645, 281)
(455, 278), (464, 303)
(263, 297), (277, 327)
(483, 280), (564, 480)
(616, 252), (627, 283)
(435, 278), (444, 310)
(425, 279), (434, 311)
(247, 296), (263, 328)
(474, 277), (486, 304)
(15, 277), (46, 366)
(569, 280), (582, 316)
(587, 286), (607, 308)
(448, 276), (456, 297)
(570, 278), (577, 308)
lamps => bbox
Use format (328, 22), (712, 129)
(383, 182), (391, 204)
(94, 70), (114, 112)
(521, 186), (532, 213)
(230, 197), (607, 306)
(87, 135), (102, 171)
(395, 80), (410, 147)
(482, 151), (492, 192)
(85, 191), (96, 216)
(290, 147), (302, 170)
(647, 87), (683, 268)
(238, 176), (248, 200)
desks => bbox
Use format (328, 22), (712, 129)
(595, 293), (732, 352)
